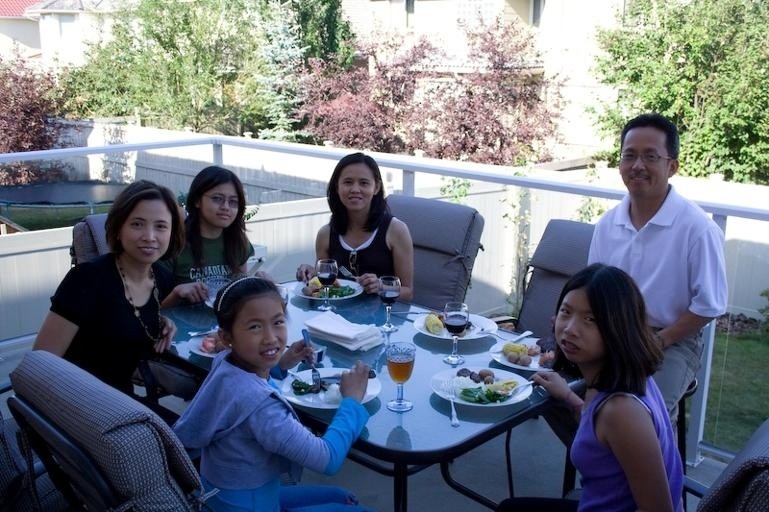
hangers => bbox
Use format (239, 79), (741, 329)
(497, 381), (537, 396)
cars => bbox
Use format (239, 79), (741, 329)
(206, 278), (232, 303)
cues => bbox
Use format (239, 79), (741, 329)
(425, 314), (443, 335)
(503, 343), (527, 355)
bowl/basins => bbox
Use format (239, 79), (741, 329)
(115, 261), (164, 343)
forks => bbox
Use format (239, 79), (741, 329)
(339, 266), (359, 280)
(302, 329), (321, 387)
(444, 380), (459, 425)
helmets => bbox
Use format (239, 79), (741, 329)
(204, 297), (216, 310)
(281, 367), (381, 410)
(293, 279), (365, 300)
(488, 338), (556, 371)
(414, 312), (498, 340)
(188, 337), (228, 358)
(430, 367), (533, 407)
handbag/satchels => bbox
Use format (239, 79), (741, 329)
(654, 331), (666, 350)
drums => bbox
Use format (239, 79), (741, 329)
(488, 330), (534, 352)
(320, 370), (377, 384)
(391, 311), (435, 314)
(306, 276), (309, 286)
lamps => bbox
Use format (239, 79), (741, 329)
(71, 206), (205, 426)
(691, 419), (768, 512)
(439, 220), (597, 511)
(7, 348), (218, 511)
(0, 384), (73, 512)
(384, 194), (485, 312)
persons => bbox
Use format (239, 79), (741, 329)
(160, 166), (274, 311)
(173, 276), (374, 512)
(499, 264), (684, 512)
(33, 178), (186, 427)
(589, 113), (730, 416)
(295, 153), (415, 305)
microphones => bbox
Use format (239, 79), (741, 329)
(678, 378), (699, 511)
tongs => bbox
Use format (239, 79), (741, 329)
(441, 376), (484, 398)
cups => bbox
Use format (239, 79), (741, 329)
(620, 149), (673, 163)
(202, 193), (240, 208)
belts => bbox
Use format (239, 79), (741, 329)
(276, 286), (289, 307)
(379, 276), (402, 333)
(386, 341), (415, 412)
(316, 259), (338, 310)
(443, 302), (468, 365)
